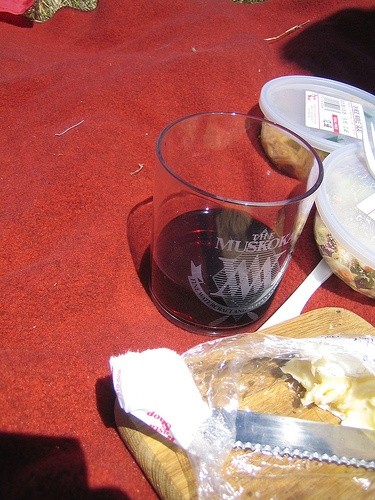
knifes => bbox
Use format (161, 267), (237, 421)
(212, 407), (375, 468)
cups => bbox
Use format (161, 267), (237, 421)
(148, 111), (324, 336)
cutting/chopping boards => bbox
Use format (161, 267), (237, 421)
(112, 307), (375, 500)
